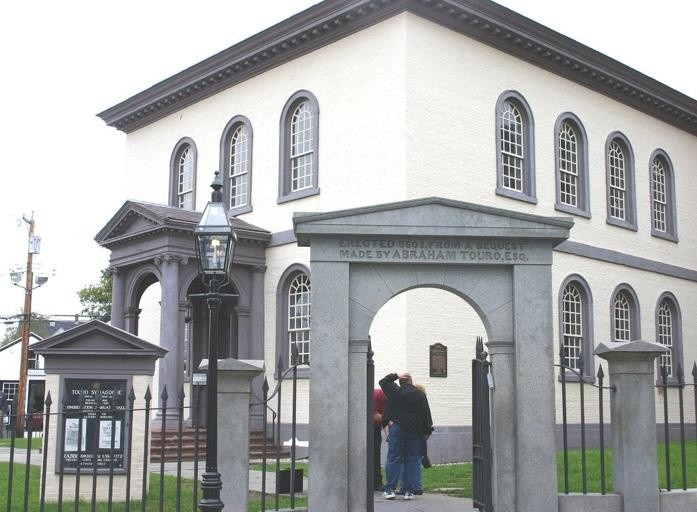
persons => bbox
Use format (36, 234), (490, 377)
(374, 372), (434, 500)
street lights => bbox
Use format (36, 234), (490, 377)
(193, 170), (237, 512)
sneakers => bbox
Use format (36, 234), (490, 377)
(382, 491), (396, 499)
(403, 491), (414, 500)
